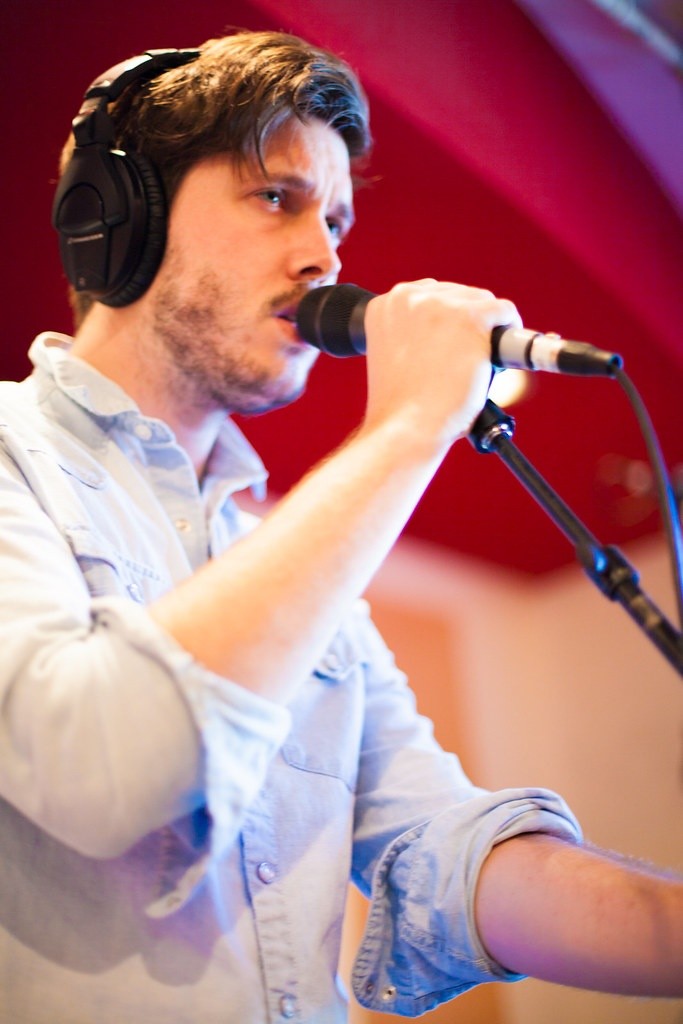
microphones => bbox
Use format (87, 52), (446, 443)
(292, 283), (625, 381)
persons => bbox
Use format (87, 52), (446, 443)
(0, 32), (681, 1023)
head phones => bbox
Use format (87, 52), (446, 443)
(51, 48), (204, 310)
